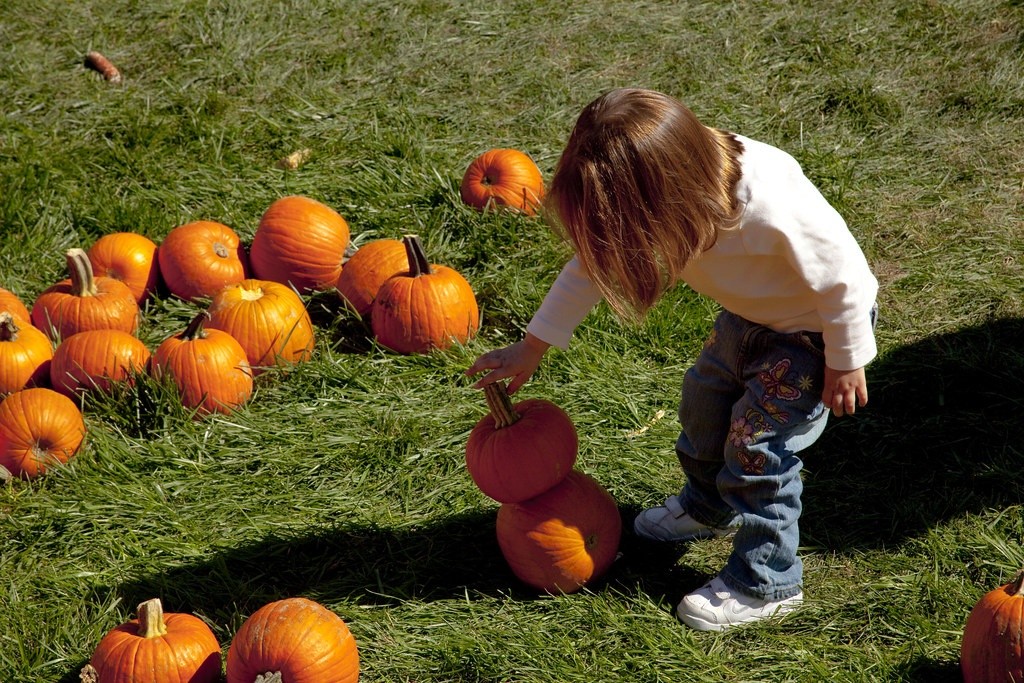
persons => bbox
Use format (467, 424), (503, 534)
(466, 87), (878, 631)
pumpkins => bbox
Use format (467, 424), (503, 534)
(1, 147), (1024, 683)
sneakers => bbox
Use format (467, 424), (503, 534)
(633, 491), (733, 542)
(676, 575), (804, 631)
